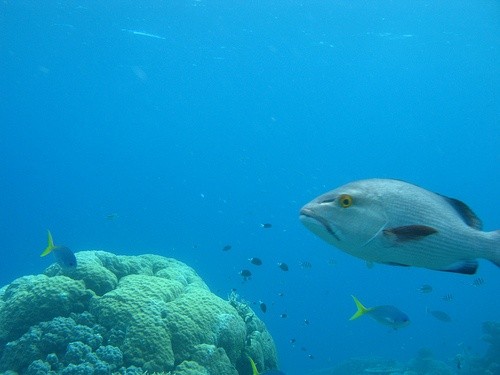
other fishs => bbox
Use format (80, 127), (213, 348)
(230, 257), (314, 360)
(471, 277), (485, 286)
(440, 294), (454, 303)
(222, 244), (231, 251)
(415, 284), (432, 294)
(243, 351), (287, 375)
(348, 295), (411, 331)
(425, 308), (451, 322)
(40, 229), (77, 274)
(297, 177), (500, 276)
(260, 223), (272, 228)
